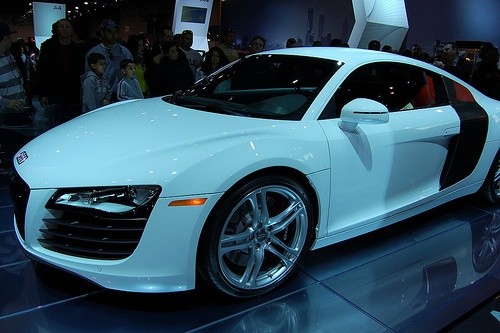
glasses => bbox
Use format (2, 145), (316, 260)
(183, 30), (192, 33)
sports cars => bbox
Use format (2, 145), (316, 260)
(12, 47), (500, 305)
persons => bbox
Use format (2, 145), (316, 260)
(287, 37), (500, 101)
(0, 19), (266, 160)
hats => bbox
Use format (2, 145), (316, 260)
(98, 19), (120, 30)
(0, 23), (18, 35)
